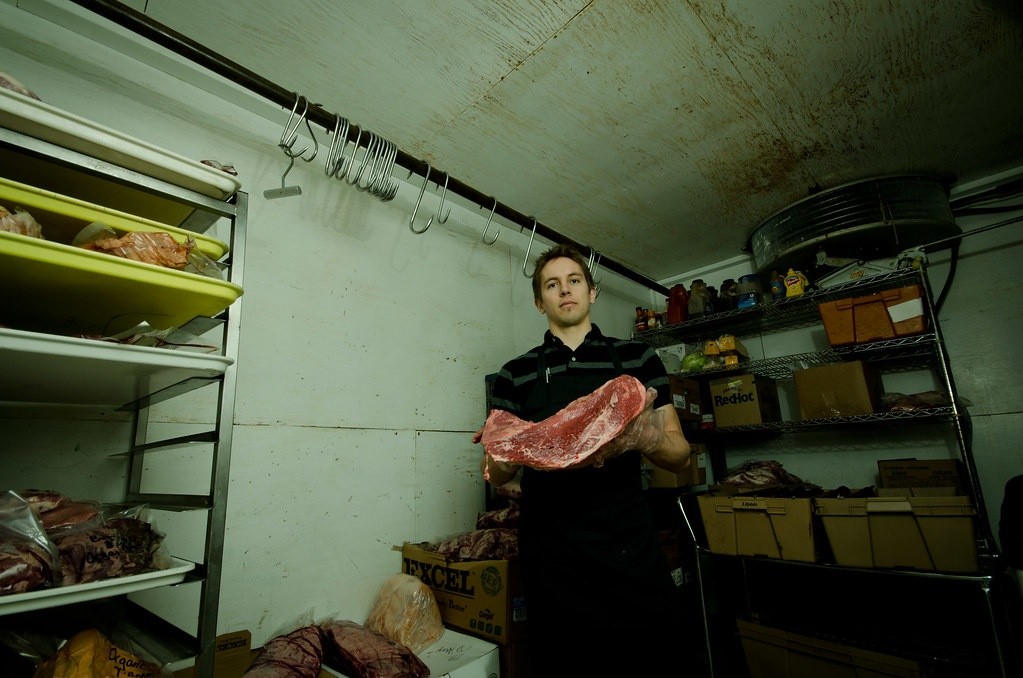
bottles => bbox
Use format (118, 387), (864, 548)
(771, 271), (785, 303)
(687, 280), (713, 318)
(784, 268), (806, 298)
(667, 284), (687, 323)
(636, 306), (663, 331)
(738, 275), (760, 309)
(719, 279), (738, 310)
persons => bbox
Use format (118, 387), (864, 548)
(480, 244), (721, 677)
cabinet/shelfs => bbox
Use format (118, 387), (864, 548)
(632, 261), (1008, 678)
(0, 129), (248, 678)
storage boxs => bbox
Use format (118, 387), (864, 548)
(816, 284), (928, 348)
(794, 359), (877, 421)
(814, 486), (979, 574)
(403, 541), (514, 678)
(710, 373), (782, 427)
(695, 494), (824, 563)
(878, 460), (961, 490)
(641, 441), (706, 488)
(162, 629), (352, 678)
(665, 376), (703, 419)
(734, 613), (929, 678)
